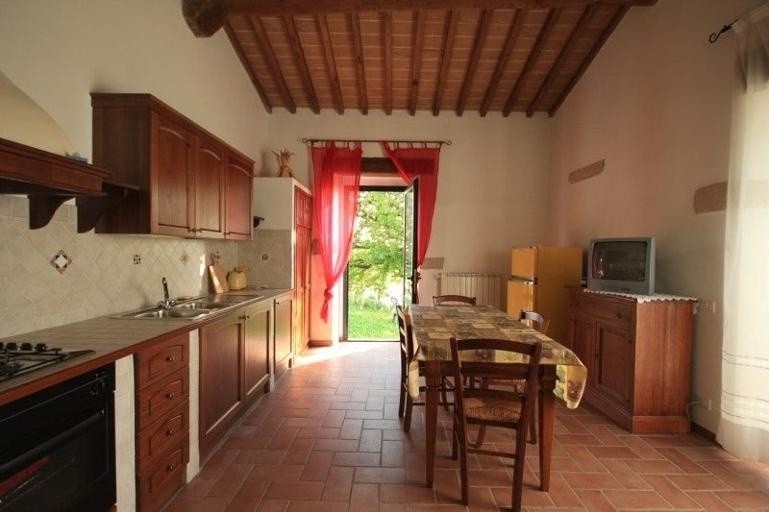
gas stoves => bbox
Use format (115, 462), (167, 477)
(0, 341), (71, 380)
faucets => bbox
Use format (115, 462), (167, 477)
(162, 276), (171, 301)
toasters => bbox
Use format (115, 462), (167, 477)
(227, 270), (248, 290)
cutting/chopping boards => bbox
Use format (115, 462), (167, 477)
(207, 252), (229, 294)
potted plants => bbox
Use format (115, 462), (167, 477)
(269, 144), (300, 177)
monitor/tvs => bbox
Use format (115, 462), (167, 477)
(588, 236), (655, 295)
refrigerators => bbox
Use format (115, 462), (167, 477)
(505, 245), (582, 340)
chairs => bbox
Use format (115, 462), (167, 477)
(432, 293), (476, 306)
(394, 304), (456, 434)
(449, 335), (541, 511)
(463, 310), (551, 450)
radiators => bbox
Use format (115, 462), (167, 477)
(439, 272), (502, 309)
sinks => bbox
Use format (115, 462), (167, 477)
(112, 305), (209, 321)
(179, 299), (227, 311)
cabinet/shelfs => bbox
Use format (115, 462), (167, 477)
(562, 284), (698, 434)
(254, 176), (312, 357)
(89, 91), (253, 243)
(273, 290), (296, 383)
(134, 328), (191, 510)
(200, 293), (274, 472)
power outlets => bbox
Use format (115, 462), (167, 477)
(695, 395), (711, 411)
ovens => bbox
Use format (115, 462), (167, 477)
(0, 361), (117, 511)
